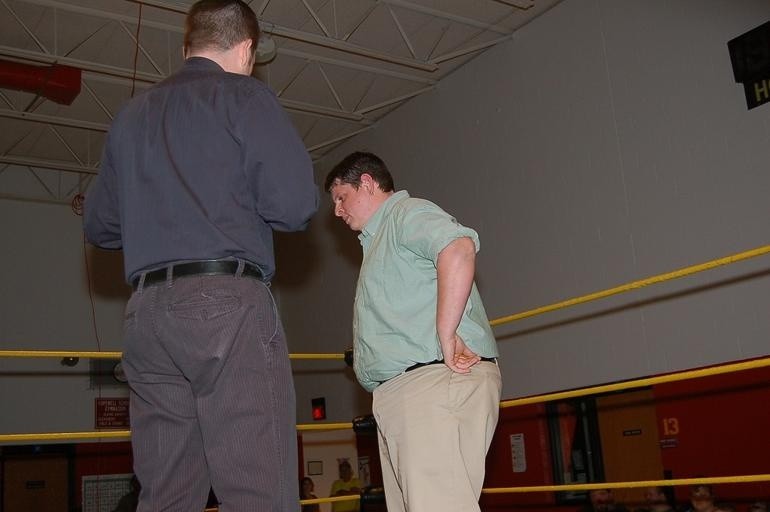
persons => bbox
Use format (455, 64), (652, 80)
(329, 461), (367, 511)
(114, 475), (140, 512)
(324, 151), (502, 511)
(584, 475), (770, 512)
(300, 477), (320, 511)
(83, 1), (319, 512)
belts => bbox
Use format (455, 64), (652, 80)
(376, 357), (497, 385)
(131, 260), (265, 291)
